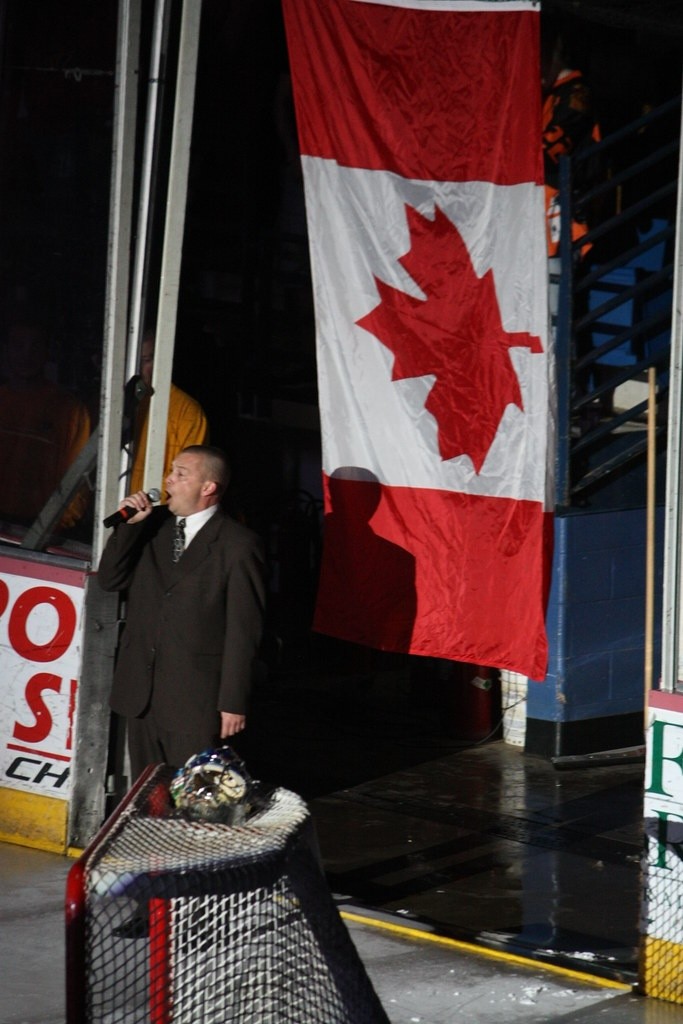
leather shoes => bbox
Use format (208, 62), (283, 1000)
(110, 918), (151, 939)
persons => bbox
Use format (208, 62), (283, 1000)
(42, 323), (205, 551)
(89, 443), (263, 937)
(0, 317), (92, 537)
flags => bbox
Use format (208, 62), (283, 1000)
(284, 0), (553, 685)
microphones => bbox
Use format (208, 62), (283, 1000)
(103, 488), (161, 528)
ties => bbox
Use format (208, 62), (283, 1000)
(171, 518), (186, 570)
